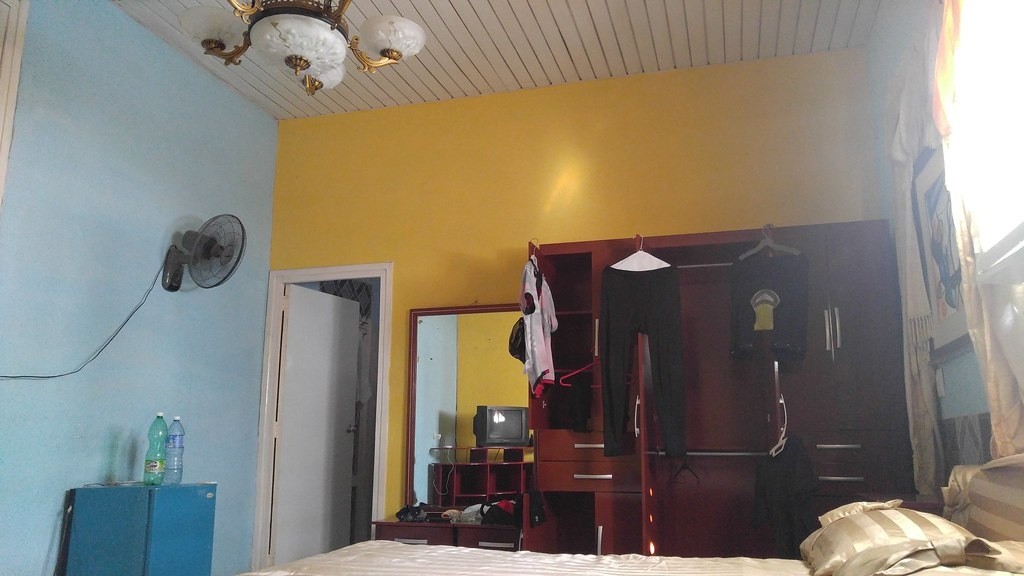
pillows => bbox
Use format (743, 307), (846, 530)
(799, 499), (1001, 575)
(941, 455), (1024, 543)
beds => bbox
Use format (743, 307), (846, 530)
(230, 456), (1024, 576)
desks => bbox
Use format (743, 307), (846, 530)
(372, 522), (520, 552)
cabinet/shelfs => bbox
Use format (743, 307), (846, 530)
(428, 446), (533, 509)
(63, 482), (216, 576)
(530, 215), (909, 559)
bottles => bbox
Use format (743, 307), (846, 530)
(143, 412), (167, 485)
(163, 415), (185, 486)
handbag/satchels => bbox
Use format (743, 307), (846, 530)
(480, 500), (517, 527)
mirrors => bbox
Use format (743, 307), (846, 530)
(406, 303), (533, 521)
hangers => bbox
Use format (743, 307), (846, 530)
(560, 349), (636, 389)
(735, 223), (806, 262)
(767, 423), (788, 456)
(632, 232), (649, 254)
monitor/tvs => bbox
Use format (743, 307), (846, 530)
(473, 406), (529, 448)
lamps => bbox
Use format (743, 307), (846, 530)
(178, 0), (425, 97)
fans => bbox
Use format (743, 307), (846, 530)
(162, 214), (247, 292)
